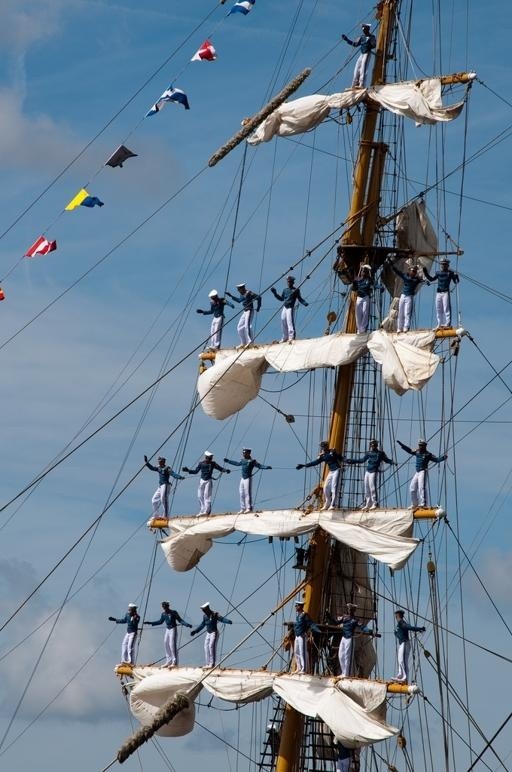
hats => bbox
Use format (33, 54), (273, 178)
(129, 602), (138, 608)
(241, 448), (251, 454)
(235, 283), (245, 290)
(162, 602), (169, 605)
(395, 610), (405, 614)
(418, 440), (427, 445)
(362, 23), (371, 27)
(204, 451), (213, 459)
(209, 289), (217, 300)
(369, 440), (378, 444)
(158, 456), (166, 460)
(439, 259), (450, 265)
(347, 603), (356, 608)
(408, 266), (418, 270)
(360, 265), (369, 271)
(200, 601), (209, 610)
(294, 601), (304, 606)
(287, 276), (294, 280)
(319, 441), (327, 447)
(267, 725), (275, 730)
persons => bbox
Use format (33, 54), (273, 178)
(292, 601), (312, 675)
(144, 601), (192, 668)
(337, 604), (372, 677)
(296, 441), (350, 511)
(190, 602), (232, 669)
(182, 451), (230, 516)
(352, 439), (393, 510)
(196, 289), (234, 349)
(336, 741), (350, 772)
(350, 264), (374, 335)
(108, 603), (141, 665)
(142, 454), (185, 520)
(270, 275), (308, 343)
(397, 438), (448, 510)
(391, 265), (430, 332)
(223, 447), (272, 514)
(341, 23), (376, 91)
(391, 609), (426, 683)
(423, 258), (455, 331)
(224, 283), (261, 348)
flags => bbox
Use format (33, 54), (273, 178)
(145, 83), (191, 118)
(190, 40), (216, 62)
(104, 144), (138, 169)
(64, 188), (104, 215)
(0, 286), (5, 302)
(227, 0), (255, 17)
(24, 235), (57, 258)
